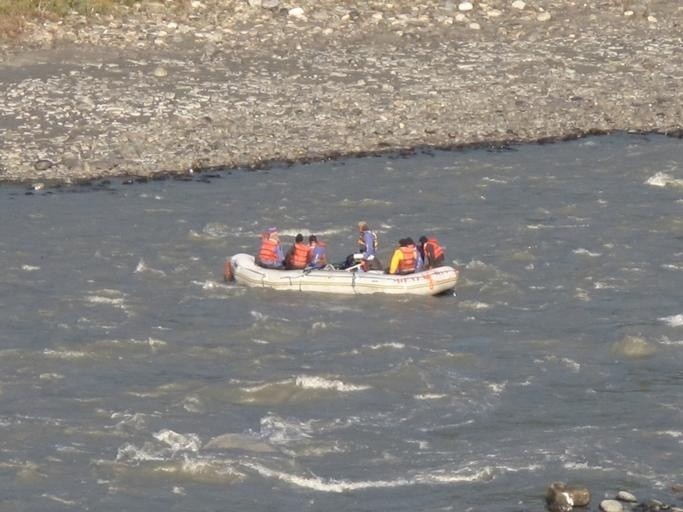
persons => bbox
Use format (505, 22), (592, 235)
(406, 237), (423, 272)
(389, 238), (417, 274)
(260, 226), (277, 242)
(283, 233), (308, 270)
(256, 232), (283, 269)
(308, 235), (328, 269)
(340, 221), (378, 269)
(420, 234), (444, 266)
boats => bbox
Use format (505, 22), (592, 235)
(222, 253), (459, 297)
